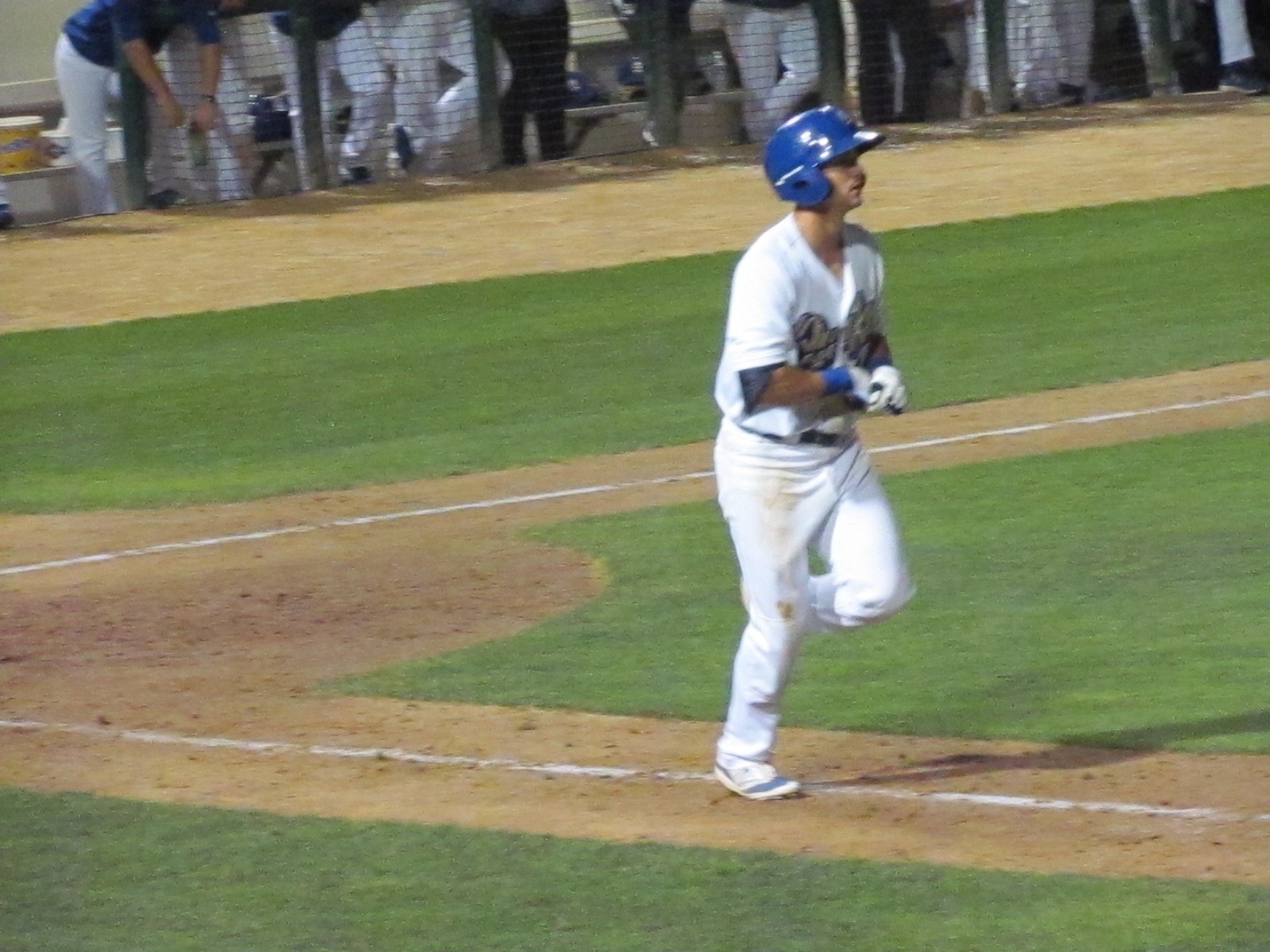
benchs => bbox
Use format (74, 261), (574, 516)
(0, 27), (754, 227)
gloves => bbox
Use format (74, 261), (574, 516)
(849, 365), (872, 404)
(866, 364), (908, 415)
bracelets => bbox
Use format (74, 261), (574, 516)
(202, 93), (216, 103)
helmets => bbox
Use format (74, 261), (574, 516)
(765, 106), (885, 207)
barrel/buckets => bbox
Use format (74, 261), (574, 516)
(0, 114), (46, 177)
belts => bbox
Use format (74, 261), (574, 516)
(740, 426), (839, 446)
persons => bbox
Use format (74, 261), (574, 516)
(51, 0), (1270, 219)
(712, 104), (920, 801)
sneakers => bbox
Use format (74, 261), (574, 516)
(714, 751), (802, 801)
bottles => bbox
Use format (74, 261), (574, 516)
(713, 50), (726, 92)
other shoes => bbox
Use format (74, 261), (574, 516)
(150, 189), (188, 208)
(722, 133), (749, 147)
(337, 162), (374, 183)
(1218, 69), (1270, 95)
(1018, 86), (1073, 110)
(384, 123), (413, 179)
(641, 126), (659, 148)
